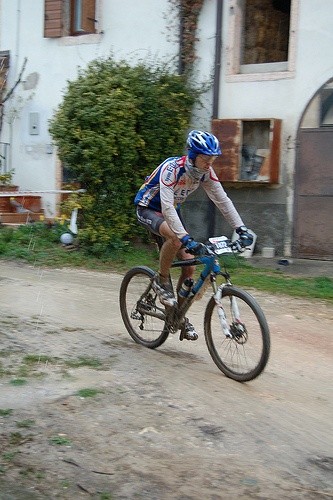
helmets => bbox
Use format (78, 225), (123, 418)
(186, 130), (223, 156)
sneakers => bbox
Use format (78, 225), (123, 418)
(179, 318), (198, 340)
(150, 271), (176, 307)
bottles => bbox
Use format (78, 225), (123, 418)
(178, 277), (194, 298)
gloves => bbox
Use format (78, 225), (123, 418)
(235, 226), (254, 245)
(181, 234), (206, 256)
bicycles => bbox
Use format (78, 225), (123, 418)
(117, 231), (273, 383)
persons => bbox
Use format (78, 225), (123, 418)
(131, 129), (253, 339)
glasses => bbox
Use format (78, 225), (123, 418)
(198, 153), (219, 161)
